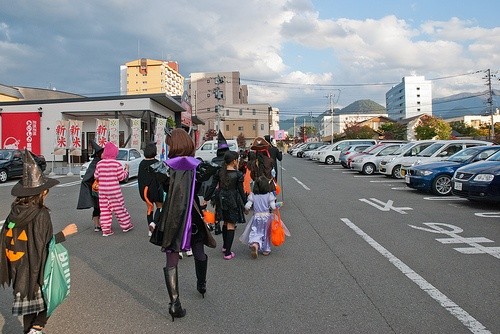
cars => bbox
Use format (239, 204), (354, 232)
(347, 145), (384, 168)
(405, 146), (500, 196)
(340, 145), (371, 168)
(0, 149), (47, 183)
(80, 148), (146, 183)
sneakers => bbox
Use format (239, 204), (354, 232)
(102, 230), (114, 237)
(123, 224), (134, 232)
(148, 230), (153, 237)
(149, 222), (157, 233)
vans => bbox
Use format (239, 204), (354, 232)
(194, 140), (238, 163)
(350, 143), (405, 175)
(378, 140), (438, 179)
(317, 139), (378, 165)
(287, 142), (330, 160)
(400, 140), (494, 179)
(450, 150), (500, 205)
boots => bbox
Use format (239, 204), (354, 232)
(195, 254), (208, 299)
(163, 266), (186, 322)
(215, 222), (222, 235)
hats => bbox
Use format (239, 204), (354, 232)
(11, 148), (60, 197)
(214, 129), (233, 150)
(89, 139), (104, 158)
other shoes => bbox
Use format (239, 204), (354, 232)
(27, 328), (42, 334)
(224, 252), (235, 260)
(263, 250), (272, 256)
(222, 248), (227, 252)
(250, 244), (259, 259)
(179, 252), (183, 259)
(186, 250), (193, 256)
(94, 226), (101, 232)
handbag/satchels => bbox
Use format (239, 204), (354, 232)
(42, 234), (71, 318)
(202, 209), (216, 224)
(276, 183), (281, 195)
(270, 209), (285, 246)
(92, 180), (98, 197)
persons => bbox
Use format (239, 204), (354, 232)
(94, 142), (134, 237)
(150, 128), (217, 322)
(244, 176), (291, 260)
(196, 129), (284, 210)
(76, 141), (105, 232)
(0, 149), (78, 334)
(138, 143), (164, 237)
(203, 151), (249, 260)
